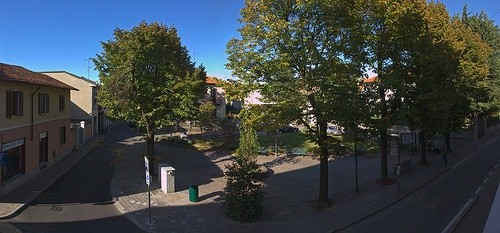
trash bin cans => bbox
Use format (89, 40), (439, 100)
(157, 162), (176, 194)
(188, 183), (198, 201)
